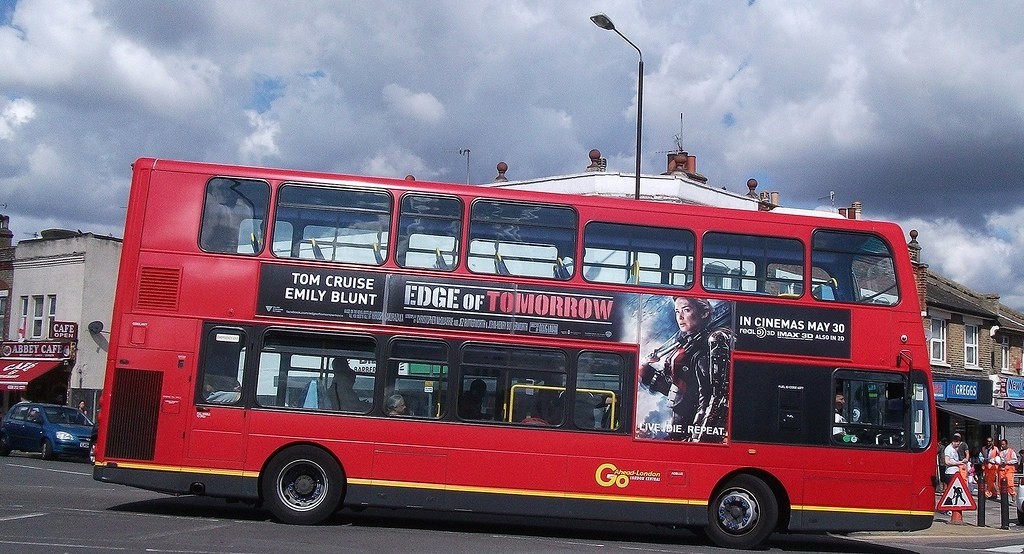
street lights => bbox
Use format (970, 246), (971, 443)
(589, 9), (644, 269)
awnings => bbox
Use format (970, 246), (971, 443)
(0, 359), (61, 391)
(935, 401), (1024, 427)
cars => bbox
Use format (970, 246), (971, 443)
(1015, 473), (1024, 526)
(0, 400), (96, 462)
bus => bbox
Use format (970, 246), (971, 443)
(87, 157), (941, 550)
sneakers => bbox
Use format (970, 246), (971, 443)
(1010, 500), (1016, 506)
(988, 494), (997, 500)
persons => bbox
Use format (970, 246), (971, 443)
(329, 355), (616, 435)
(639, 296), (729, 441)
(76, 400), (88, 425)
(937, 432), (1024, 502)
(18, 394), (30, 404)
(833, 391), (872, 444)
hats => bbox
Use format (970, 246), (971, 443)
(386, 394), (403, 408)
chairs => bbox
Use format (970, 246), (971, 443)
(301, 380), (340, 410)
(827, 278), (849, 302)
(628, 261), (640, 285)
(436, 247), (449, 270)
(494, 252), (510, 275)
(553, 257), (571, 279)
(250, 233), (260, 252)
(374, 243), (385, 264)
(311, 238), (325, 260)
